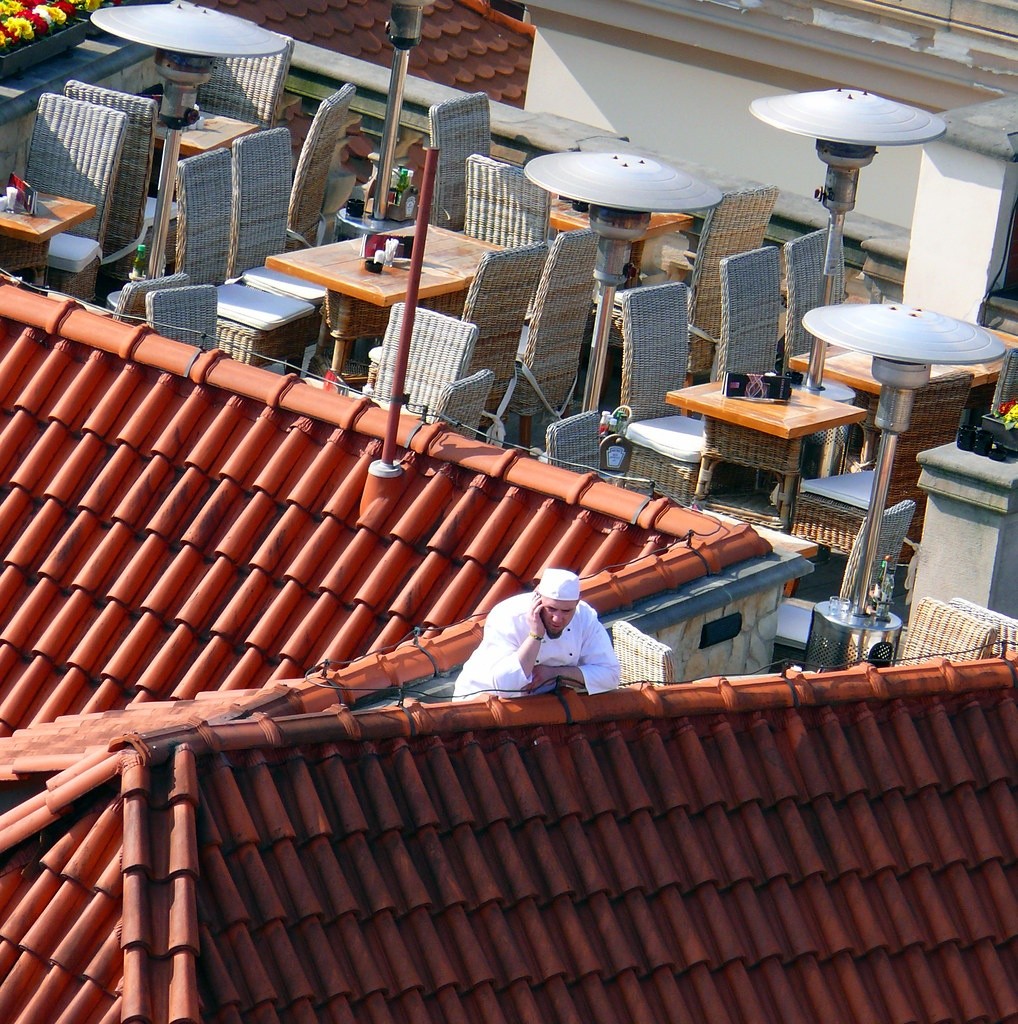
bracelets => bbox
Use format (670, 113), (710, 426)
(529, 631), (543, 640)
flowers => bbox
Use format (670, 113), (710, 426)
(990, 399), (1018, 430)
(0, 0), (130, 56)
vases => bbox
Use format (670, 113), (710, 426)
(74, 3), (127, 39)
(979, 413), (1018, 452)
(0, 17), (91, 82)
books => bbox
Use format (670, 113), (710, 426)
(9, 172), (39, 215)
(722, 371), (792, 400)
(359, 233), (414, 259)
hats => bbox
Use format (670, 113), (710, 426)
(539, 568), (579, 600)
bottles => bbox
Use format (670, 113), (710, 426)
(866, 554), (895, 621)
(373, 250), (385, 266)
(599, 411), (628, 447)
(389, 167), (414, 207)
(131, 244), (147, 280)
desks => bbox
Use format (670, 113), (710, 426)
(265, 222), (512, 385)
(0, 192), (98, 288)
(546, 191), (693, 291)
(788, 324), (1018, 467)
(152, 111), (258, 197)
(664, 374), (867, 534)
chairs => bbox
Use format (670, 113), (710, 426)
(0, 37), (1018, 685)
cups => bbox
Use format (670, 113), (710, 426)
(5, 187), (19, 213)
(384, 248), (396, 267)
(829, 596), (850, 620)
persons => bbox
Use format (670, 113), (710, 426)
(450, 567), (620, 703)
